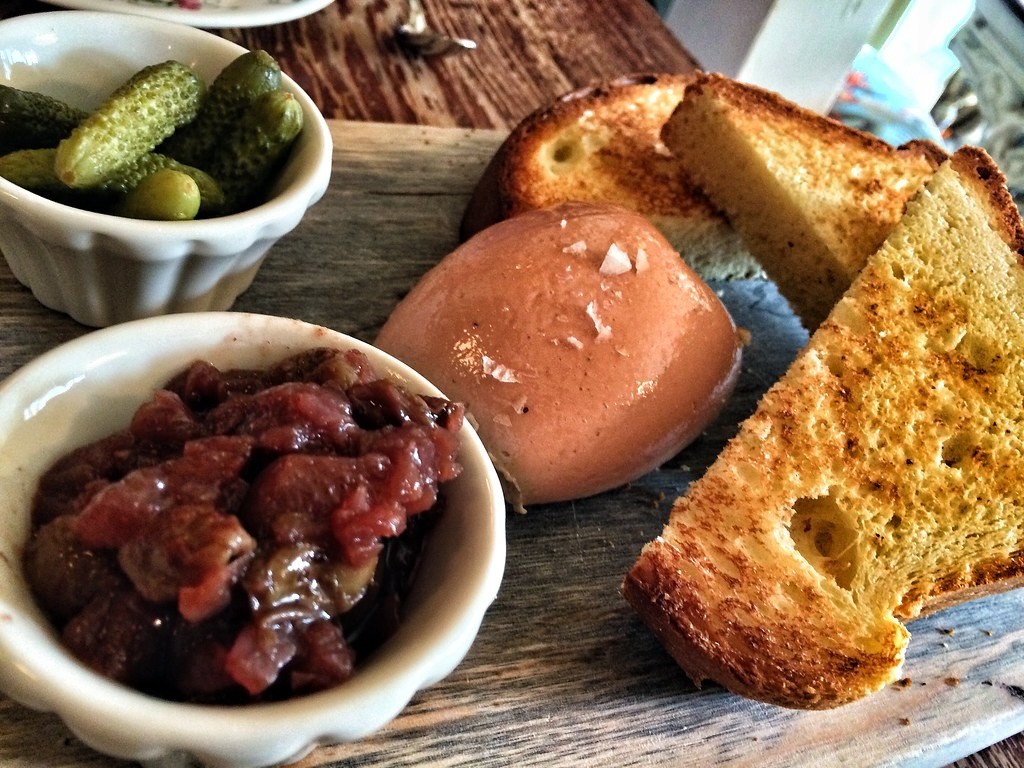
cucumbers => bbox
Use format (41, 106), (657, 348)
(0, 50), (304, 221)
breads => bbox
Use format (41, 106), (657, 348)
(373, 72), (1024, 711)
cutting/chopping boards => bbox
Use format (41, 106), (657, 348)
(0, 124), (1023, 768)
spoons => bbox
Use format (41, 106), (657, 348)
(395, 1), (477, 58)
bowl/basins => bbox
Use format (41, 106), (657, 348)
(0, 311), (509, 764)
(0, 11), (334, 327)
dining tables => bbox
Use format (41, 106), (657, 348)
(0, 0), (1024, 768)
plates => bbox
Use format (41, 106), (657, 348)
(44, 1), (334, 28)
